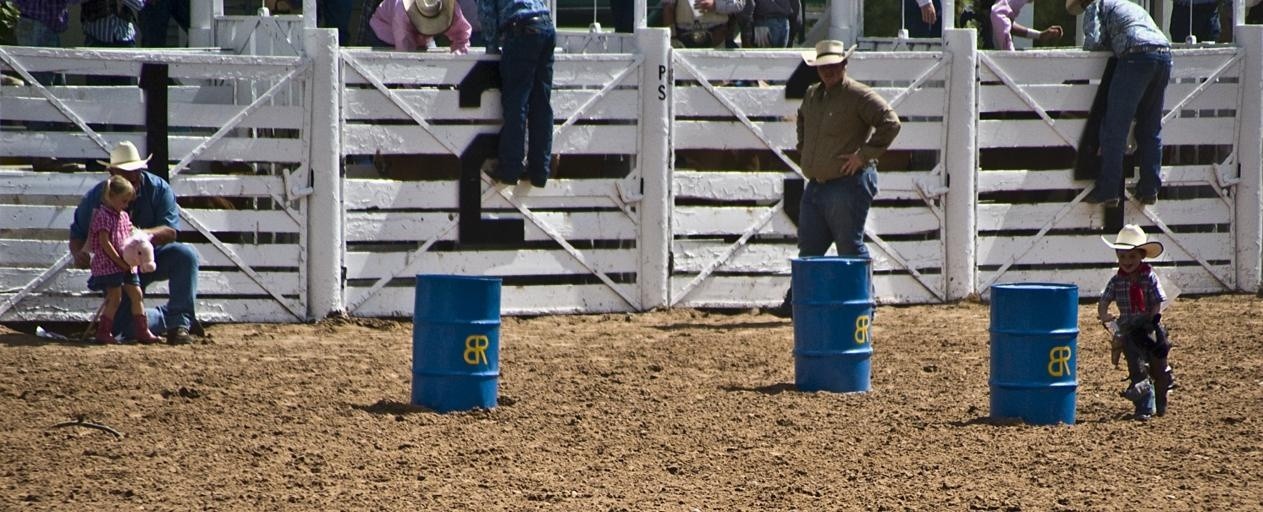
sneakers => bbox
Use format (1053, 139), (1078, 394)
(519, 172), (547, 189)
(189, 316), (208, 339)
(1103, 198), (1121, 209)
(1133, 391), (1170, 422)
(483, 169), (518, 187)
(1131, 192), (1158, 205)
(760, 287), (794, 318)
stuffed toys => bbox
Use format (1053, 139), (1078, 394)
(120, 231), (157, 275)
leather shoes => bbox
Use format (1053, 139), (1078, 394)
(166, 325), (192, 346)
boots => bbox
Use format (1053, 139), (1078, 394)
(131, 310), (161, 345)
(95, 313), (117, 345)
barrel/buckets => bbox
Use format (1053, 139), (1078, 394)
(989, 279), (1080, 422)
(411, 271), (501, 410)
(792, 257), (874, 391)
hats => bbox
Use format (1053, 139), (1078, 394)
(1065, 0), (1090, 16)
(1098, 222), (1162, 258)
(796, 38), (858, 67)
(94, 138), (154, 171)
(401, 0), (458, 38)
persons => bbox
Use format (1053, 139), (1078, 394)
(12, 1), (107, 173)
(86, 175), (162, 344)
(768, 41), (900, 319)
(80, 1), (144, 173)
(368, 0), (471, 178)
(662, 0), (804, 171)
(477, 0), (556, 188)
(1097, 224), (1173, 420)
(69, 141), (204, 345)
